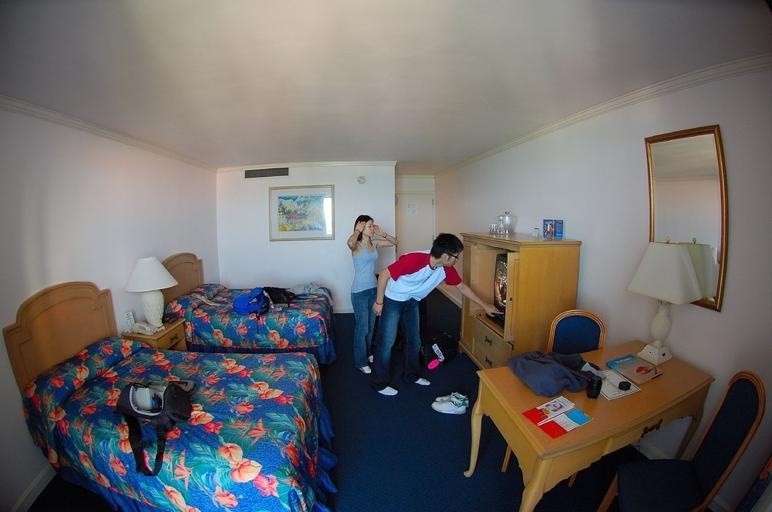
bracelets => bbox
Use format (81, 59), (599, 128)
(375, 300), (384, 305)
(383, 233), (387, 238)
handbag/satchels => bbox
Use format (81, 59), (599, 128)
(263, 287), (296, 303)
(117, 380), (195, 429)
(234, 288), (269, 316)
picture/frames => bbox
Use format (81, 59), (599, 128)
(269, 184), (335, 240)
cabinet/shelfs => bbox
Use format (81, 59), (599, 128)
(457, 232), (582, 370)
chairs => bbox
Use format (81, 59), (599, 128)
(499, 310), (606, 488)
(599, 371), (766, 512)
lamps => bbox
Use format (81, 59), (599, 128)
(626, 236), (701, 365)
(123, 257), (178, 328)
(683, 238), (716, 300)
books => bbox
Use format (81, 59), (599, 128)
(608, 354), (664, 385)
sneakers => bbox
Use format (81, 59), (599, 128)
(436, 392), (469, 406)
(432, 401), (466, 414)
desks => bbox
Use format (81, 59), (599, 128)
(464, 339), (716, 512)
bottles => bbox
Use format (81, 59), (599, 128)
(505, 209), (512, 235)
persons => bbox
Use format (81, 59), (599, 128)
(543, 222), (554, 240)
(367, 232), (502, 396)
(347, 215), (399, 373)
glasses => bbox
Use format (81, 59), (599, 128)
(446, 251), (458, 259)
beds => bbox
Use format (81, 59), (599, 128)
(2, 282), (338, 512)
(157, 253), (337, 362)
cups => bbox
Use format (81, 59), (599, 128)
(530, 226), (542, 240)
(488, 223), (499, 234)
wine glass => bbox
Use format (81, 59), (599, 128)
(497, 215), (506, 234)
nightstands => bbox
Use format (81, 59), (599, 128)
(122, 318), (187, 352)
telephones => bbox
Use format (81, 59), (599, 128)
(133, 322), (157, 335)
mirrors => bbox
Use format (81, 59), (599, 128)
(644, 125), (728, 312)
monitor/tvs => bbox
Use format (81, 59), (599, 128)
(493, 254), (506, 317)
